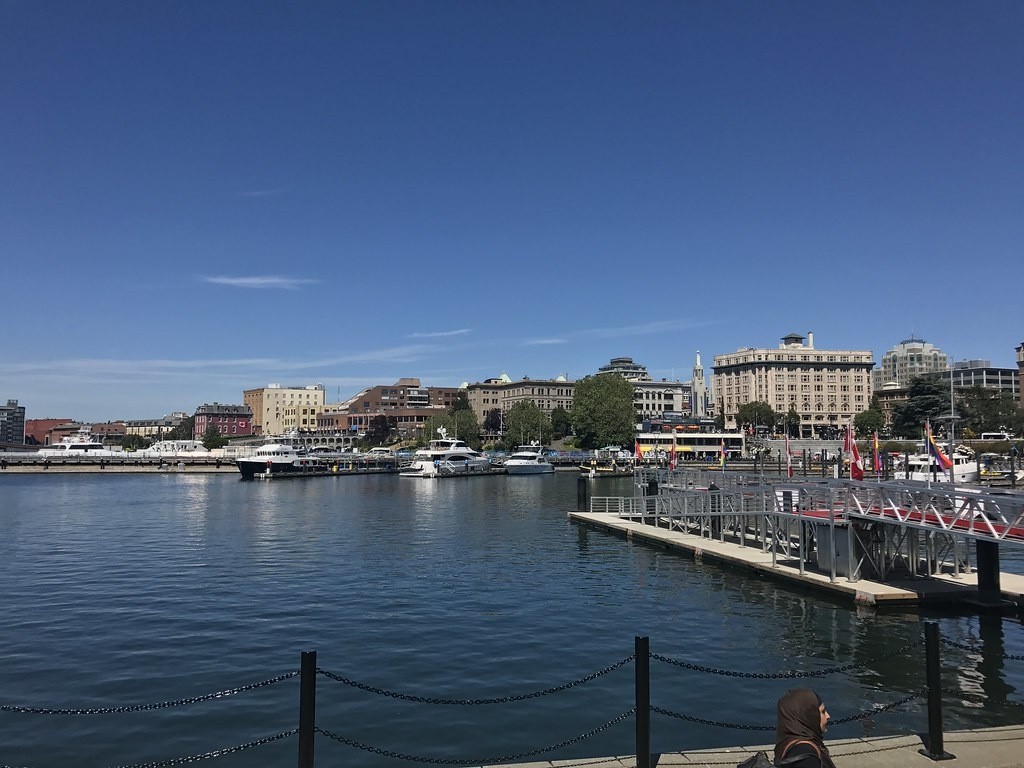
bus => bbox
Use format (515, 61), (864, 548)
(635, 433), (744, 461)
(982, 433), (1008, 440)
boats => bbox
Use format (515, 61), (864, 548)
(399, 425), (491, 476)
(37, 426), (107, 453)
(503, 446), (556, 473)
(234, 443), (327, 480)
(894, 443), (985, 483)
(979, 453), (1024, 480)
(578, 460), (670, 478)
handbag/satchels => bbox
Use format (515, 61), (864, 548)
(736, 739), (822, 768)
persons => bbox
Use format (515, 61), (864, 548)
(774, 688), (837, 768)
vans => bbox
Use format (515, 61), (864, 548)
(367, 447), (393, 456)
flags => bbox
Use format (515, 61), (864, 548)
(874, 432), (882, 477)
(670, 440), (677, 473)
(720, 440), (727, 468)
(925, 422), (952, 478)
(844, 422), (863, 481)
(786, 434), (793, 477)
(635, 441), (643, 461)
